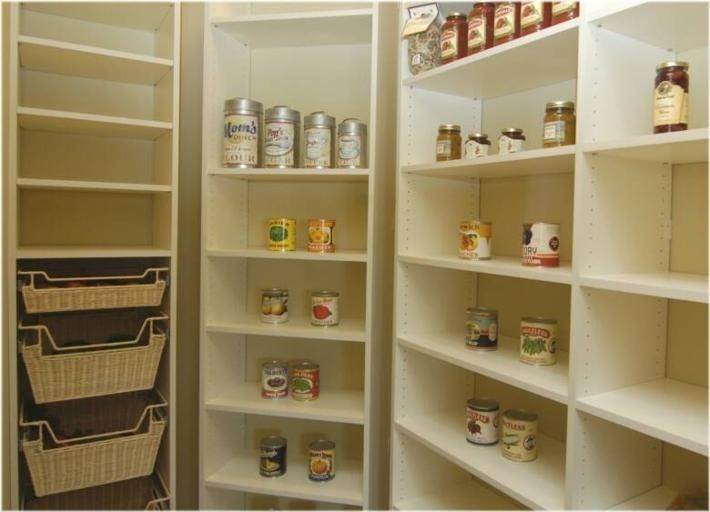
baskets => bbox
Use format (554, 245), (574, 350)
(21, 278), (166, 315)
(22, 325), (165, 405)
(20, 394), (168, 497)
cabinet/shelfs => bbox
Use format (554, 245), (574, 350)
(5, 2), (179, 510)
(390, 3), (710, 510)
(199, 1), (378, 509)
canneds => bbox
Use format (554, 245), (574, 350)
(465, 398), (500, 446)
(518, 316), (559, 366)
(310, 290), (341, 326)
(258, 434), (288, 478)
(651, 61), (691, 132)
(462, 305), (499, 350)
(221, 96), (365, 169)
(260, 288), (289, 323)
(521, 220), (561, 268)
(502, 409), (537, 462)
(267, 218), (297, 251)
(259, 358), (320, 401)
(456, 220), (493, 260)
(434, 124), (460, 161)
(543, 99), (575, 148)
(498, 127), (527, 154)
(307, 439), (336, 481)
(440, 0), (579, 64)
(306, 218), (336, 253)
(462, 132), (491, 159)
